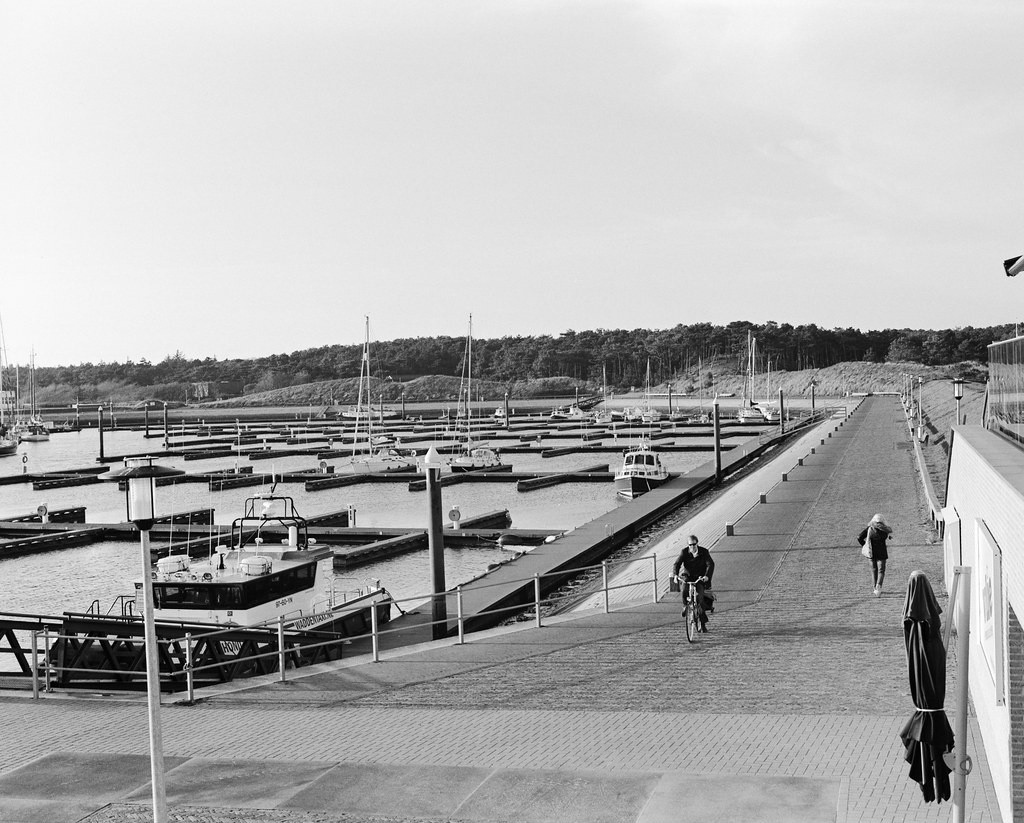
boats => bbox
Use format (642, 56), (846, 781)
(34, 494), (392, 692)
(548, 353), (702, 422)
(613, 431), (671, 499)
(333, 445), (421, 476)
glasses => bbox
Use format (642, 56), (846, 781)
(689, 543), (697, 546)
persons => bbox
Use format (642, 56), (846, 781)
(856, 513), (893, 599)
(671, 535), (715, 633)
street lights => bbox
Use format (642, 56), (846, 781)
(950, 378), (965, 427)
(902, 372), (923, 438)
(96, 456), (185, 823)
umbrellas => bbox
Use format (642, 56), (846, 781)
(896, 566), (975, 822)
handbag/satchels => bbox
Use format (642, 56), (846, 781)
(862, 526), (872, 559)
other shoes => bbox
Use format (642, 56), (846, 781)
(680, 608), (687, 618)
(700, 625), (709, 633)
(871, 584), (883, 597)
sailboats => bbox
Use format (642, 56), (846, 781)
(446, 312), (513, 472)
(737, 329), (781, 425)
(338, 315), (399, 420)
(0, 343), (72, 458)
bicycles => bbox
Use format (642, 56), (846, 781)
(677, 576), (702, 642)
